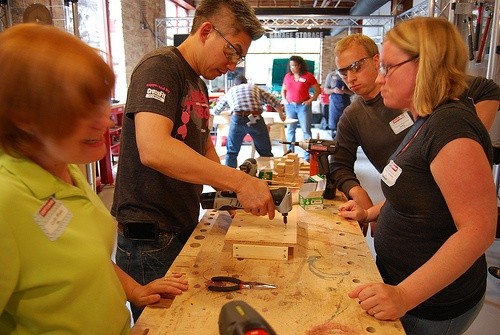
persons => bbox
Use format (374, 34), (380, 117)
(110, 0), (275, 324)
(330, 33), (500, 237)
(281, 56), (354, 161)
(0, 23), (189, 335)
(210, 74), (286, 168)
(338, 16), (498, 335)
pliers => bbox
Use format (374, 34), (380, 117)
(208, 276), (276, 291)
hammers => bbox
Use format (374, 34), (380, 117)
(476, 6), (495, 63)
(462, 14), (474, 61)
(475, 1), (488, 51)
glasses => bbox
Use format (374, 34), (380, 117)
(290, 65), (296, 69)
(337, 57), (373, 78)
(212, 25), (246, 65)
(379, 55), (420, 76)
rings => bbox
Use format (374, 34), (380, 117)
(164, 277), (167, 280)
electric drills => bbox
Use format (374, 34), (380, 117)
(279, 139), (336, 199)
(200, 187), (292, 229)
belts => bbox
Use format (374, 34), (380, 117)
(232, 110), (261, 115)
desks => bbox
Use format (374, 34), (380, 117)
(249, 112), (299, 158)
(127, 171), (406, 335)
